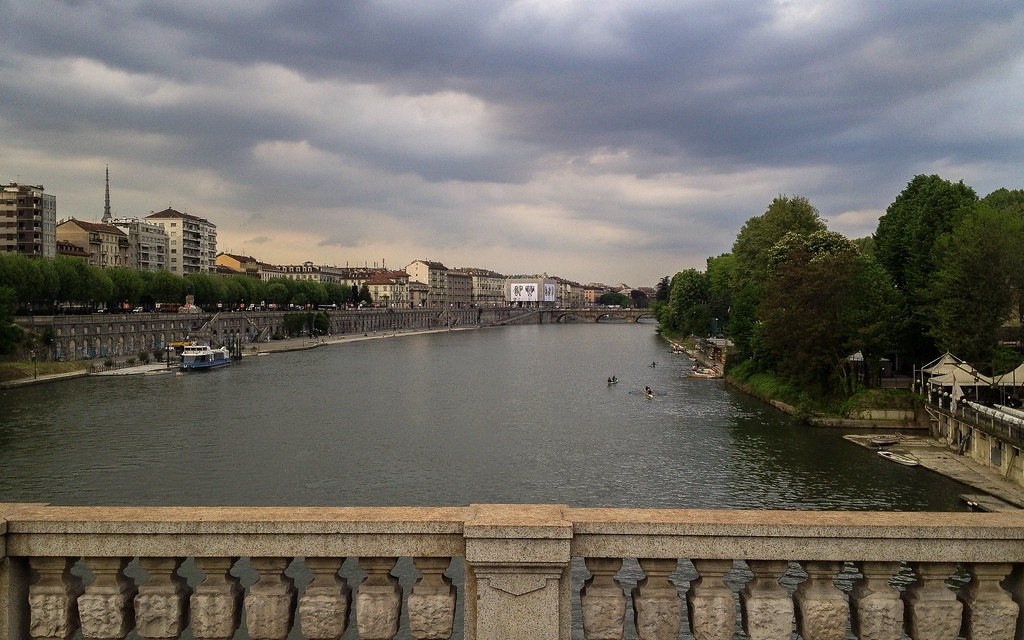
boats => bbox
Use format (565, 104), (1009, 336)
(178, 338), (237, 372)
(640, 388), (654, 400)
(877, 451), (919, 467)
(608, 379), (619, 385)
(869, 435), (900, 447)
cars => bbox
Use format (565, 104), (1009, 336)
(160, 307), (168, 313)
(222, 302), (305, 313)
(132, 306), (144, 313)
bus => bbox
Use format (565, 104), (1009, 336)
(318, 305), (338, 312)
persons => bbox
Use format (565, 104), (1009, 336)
(329, 333), (332, 338)
(645, 386), (652, 394)
(651, 361), (659, 367)
(607, 376), (616, 383)
